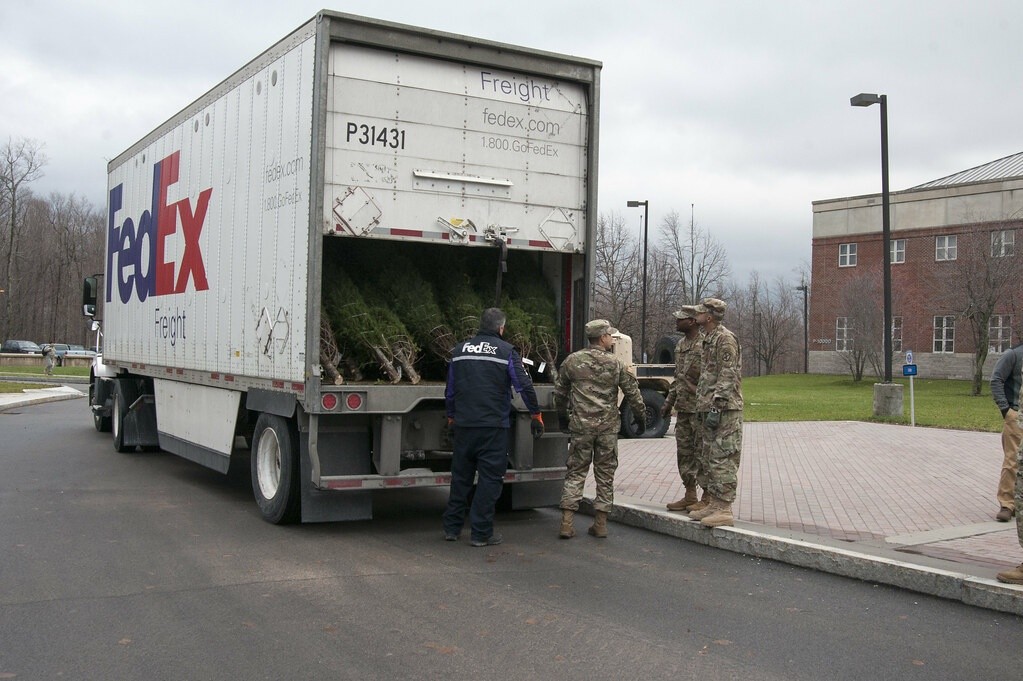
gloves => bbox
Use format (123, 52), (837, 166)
(660, 403), (674, 419)
(446, 418), (456, 444)
(530, 413), (545, 441)
(705, 406), (720, 428)
(631, 418), (646, 436)
(557, 410), (572, 434)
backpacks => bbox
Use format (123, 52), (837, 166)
(42, 345), (51, 356)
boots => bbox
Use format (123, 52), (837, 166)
(560, 509), (576, 538)
(588, 510), (608, 538)
(701, 501), (734, 527)
(689, 502), (717, 521)
(666, 485), (697, 510)
(686, 489), (715, 513)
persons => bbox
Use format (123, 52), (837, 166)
(996, 368), (1023, 584)
(687, 298), (743, 526)
(991, 346), (1023, 522)
(553, 319), (648, 540)
(660, 304), (713, 512)
(442, 308), (545, 547)
(44, 342), (58, 376)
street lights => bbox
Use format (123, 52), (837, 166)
(797, 287), (807, 373)
(626, 200), (648, 364)
(850, 94), (894, 383)
(752, 313), (762, 377)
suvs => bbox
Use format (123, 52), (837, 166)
(0, 340), (42, 354)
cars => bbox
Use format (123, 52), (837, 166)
(68, 345), (102, 357)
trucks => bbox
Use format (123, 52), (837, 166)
(80, 9), (603, 526)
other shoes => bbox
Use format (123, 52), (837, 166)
(44, 369), (53, 375)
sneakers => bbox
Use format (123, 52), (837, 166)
(996, 506), (1012, 521)
(444, 528), (462, 540)
(470, 533), (503, 546)
(996, 563), (1023, 585)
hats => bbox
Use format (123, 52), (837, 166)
(672, 304), (699, 320)
(694, 298), (726, 317)
(584, 319), (618, 338)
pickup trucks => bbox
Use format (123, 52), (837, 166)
(38, 344), (86, 366)
(609, 334), (677, 437)
